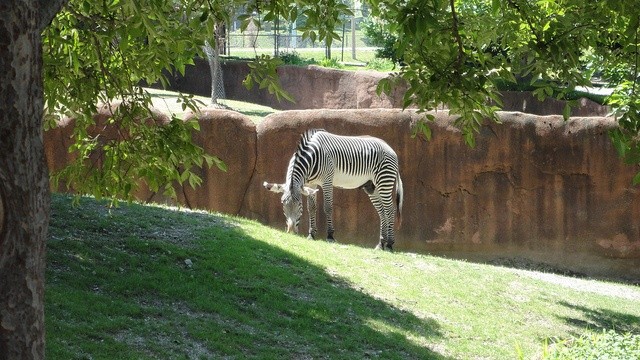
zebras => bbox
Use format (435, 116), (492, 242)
(262, 128), (404, 253)
